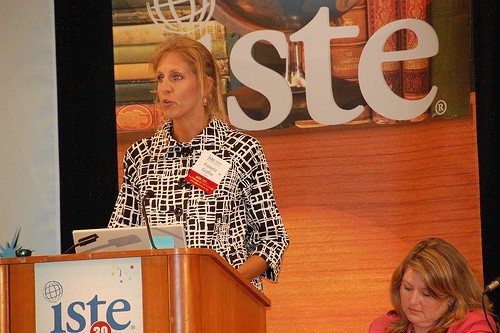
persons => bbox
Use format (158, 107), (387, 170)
(365, 236), (500, 333)
(108, 36), (290, 297)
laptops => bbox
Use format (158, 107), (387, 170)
(72, 224), (189, 254)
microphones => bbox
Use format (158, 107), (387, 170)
(142, 190), (158, 249)
(64, 234), (98, 253)
